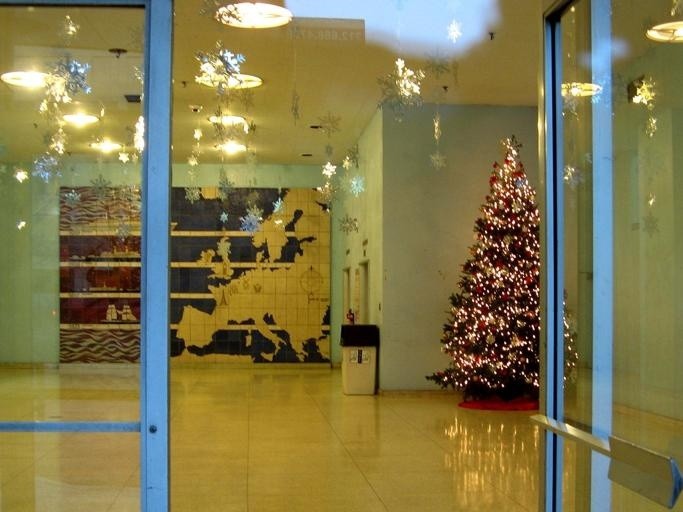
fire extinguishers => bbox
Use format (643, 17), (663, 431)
(346, 309), (354, 325)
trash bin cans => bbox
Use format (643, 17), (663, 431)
(341, 324), (378, 395)
